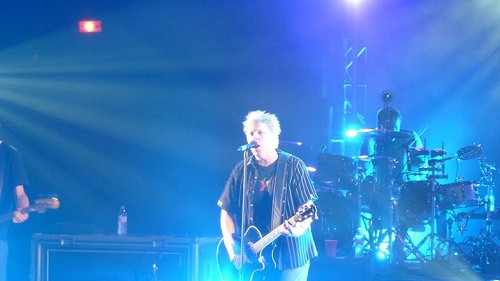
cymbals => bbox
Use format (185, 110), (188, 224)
(359, 154), (393, 160)
(357, 129), (414, 138)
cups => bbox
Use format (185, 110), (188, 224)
(324, 240), (338, 259)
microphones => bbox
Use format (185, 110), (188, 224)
(395, 234), (407, 248)
(319, 144), (326, 154)
(406, 148), (412, 172)
(382, 90), (392, 111)
(236, 141), (257, 152)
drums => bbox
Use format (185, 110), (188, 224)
(314, 154), (358, 187)
(309, 190), (353, 249)
(370, 198), (400, 228)
(439, 182), (477, 209)
(396, 181), (442, 219)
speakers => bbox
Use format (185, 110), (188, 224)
(31, 232), (194, 281)
(196, 237), (223, 281)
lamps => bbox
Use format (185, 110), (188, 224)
(74, 15), (113, 35)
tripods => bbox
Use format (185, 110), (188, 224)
(360, 138), (500, 278)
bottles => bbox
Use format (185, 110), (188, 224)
(117, 205), (128, 236)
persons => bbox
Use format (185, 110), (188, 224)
(0, 138), (30, 281)
(359, 107), (428, 262)
(217, 109), (319, 281)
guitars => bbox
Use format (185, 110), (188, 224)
(0, 196), (61, 226)
(215, 198), (318, 281)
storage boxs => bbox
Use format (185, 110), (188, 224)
(30, 232), (196, 281)
